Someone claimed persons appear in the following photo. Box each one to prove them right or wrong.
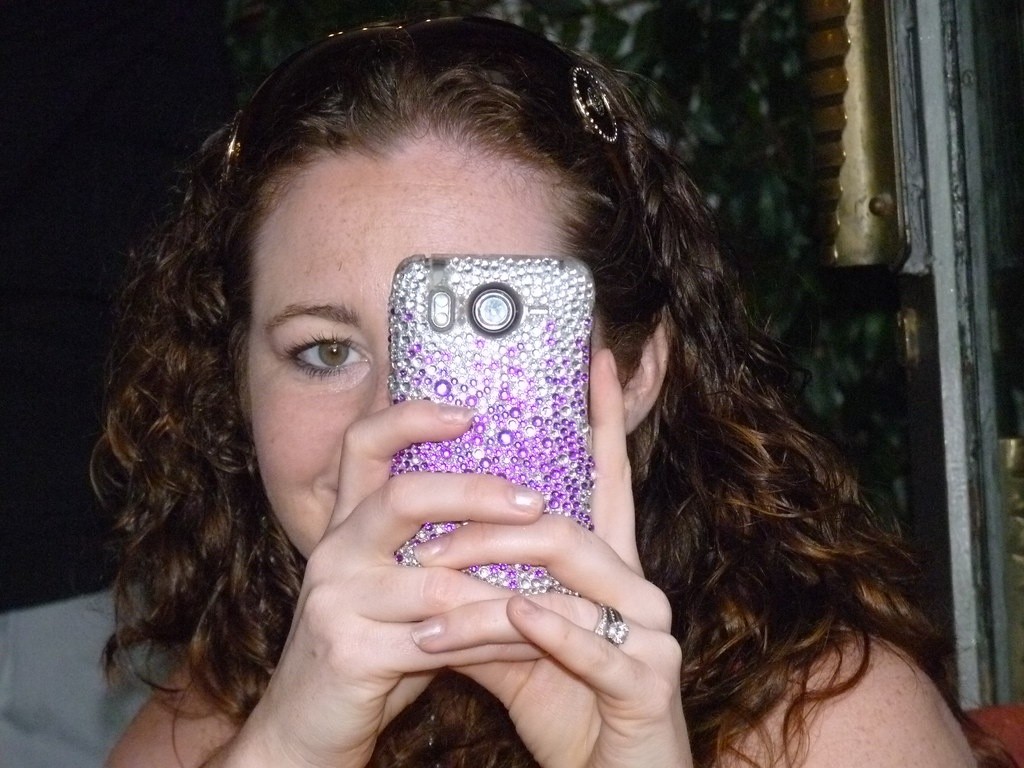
[87,17,979,767]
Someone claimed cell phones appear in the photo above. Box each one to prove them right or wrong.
[387,253,605,640]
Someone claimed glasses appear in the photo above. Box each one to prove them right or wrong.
[223,13,639,227]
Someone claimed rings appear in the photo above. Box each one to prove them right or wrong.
[593,600,629,648]
